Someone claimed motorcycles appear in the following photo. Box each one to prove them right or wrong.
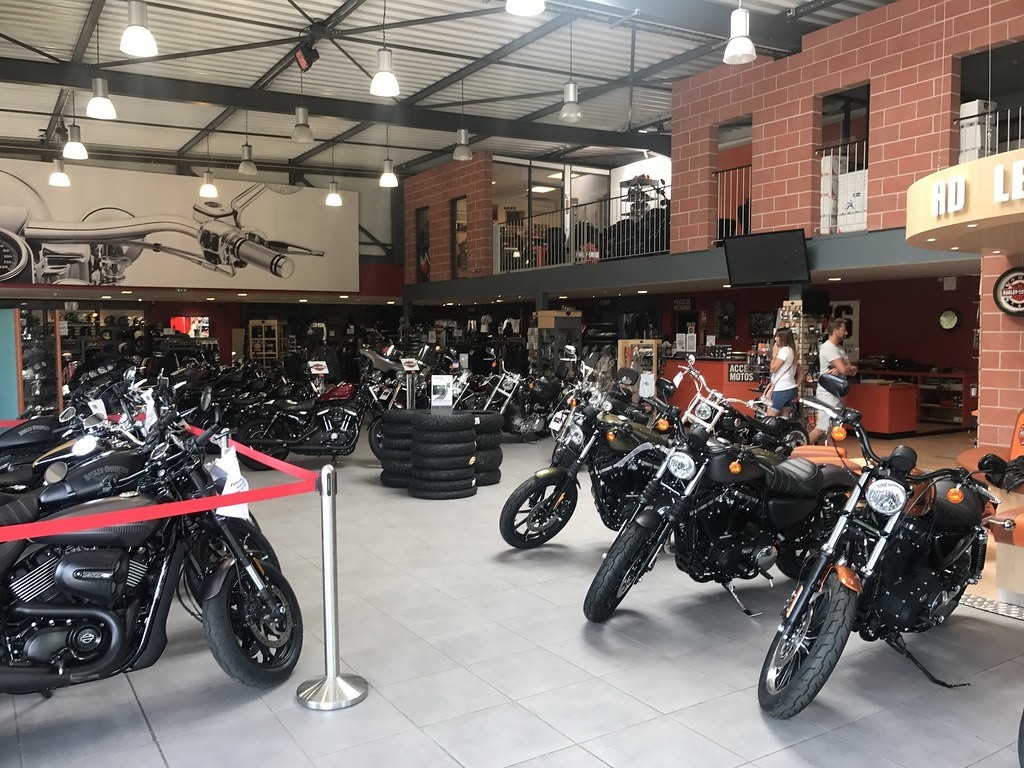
[757,372,1017,720]
[498,344,810,551]
[0,335,600,703]
[0,184,327,288]
[582,352,861,623]
[574,178,671,261]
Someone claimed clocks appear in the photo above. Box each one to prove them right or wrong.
[937,307,961,332]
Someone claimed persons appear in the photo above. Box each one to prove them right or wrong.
[767,327,804,417]
[809,318,857,445]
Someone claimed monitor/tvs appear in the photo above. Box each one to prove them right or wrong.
[723,228,810,288]
[171,316,210,339]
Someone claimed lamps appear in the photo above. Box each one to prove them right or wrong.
[291,70,314,145]
[369,0,400,97]
[295,47,319,72]
[47,25,118,188]
[199,135,218,199]
[325,146,342,206]
[379,124,398,188]
[119,1,158,57]
[504,0,545,17]
[559,21,581,122]
[452,77,475,163]
[238,110,258,176]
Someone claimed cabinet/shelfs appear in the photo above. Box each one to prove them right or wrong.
[857,368,977,429]
[248,319,288,363]
[537,310,582,382]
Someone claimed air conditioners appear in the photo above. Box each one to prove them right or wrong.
[721,0,757,65]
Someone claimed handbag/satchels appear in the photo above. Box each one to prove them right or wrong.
[764,382,776,398]
